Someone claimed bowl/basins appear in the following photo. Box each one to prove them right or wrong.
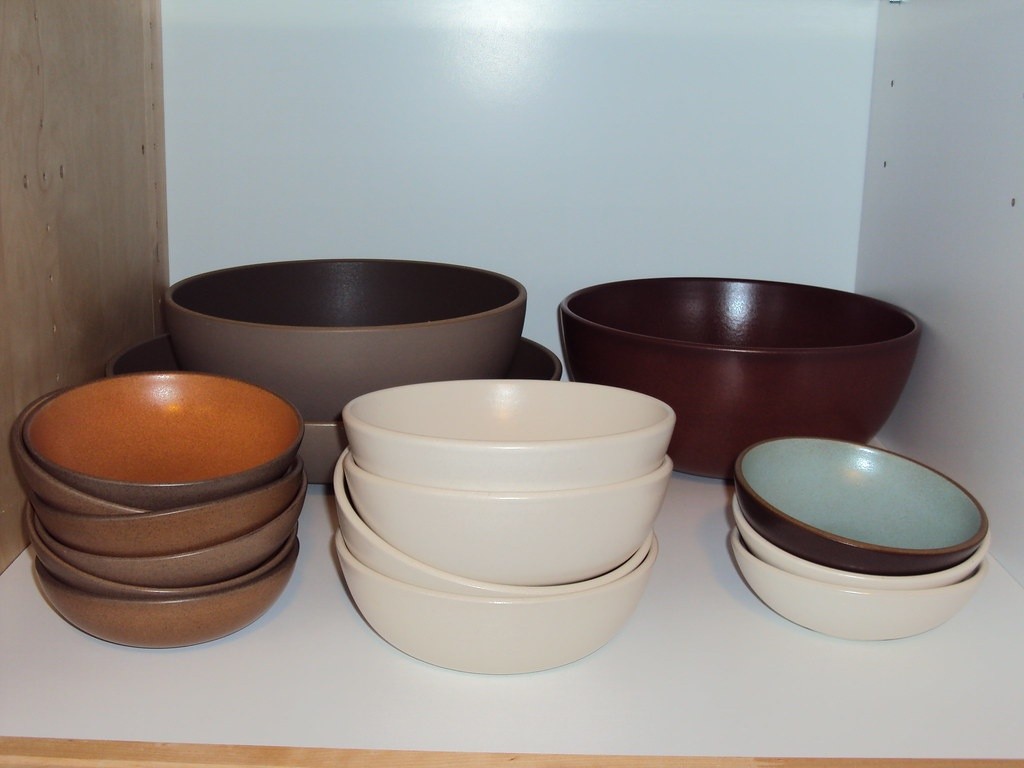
[107,339,562,483]
[162,260,527,423]
[332,379,676,676]
[558,277,919,478]
[8,369,306,648]
[729,437,988,640]
